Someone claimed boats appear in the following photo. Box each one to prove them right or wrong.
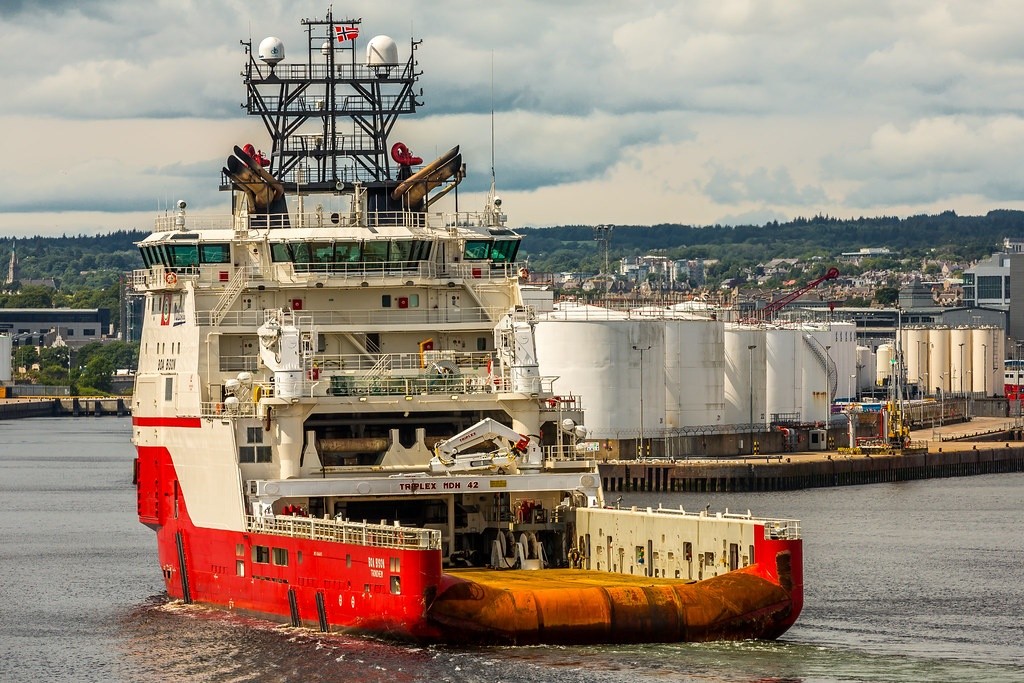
[133,4,803,644]
[1004,359,1024,406]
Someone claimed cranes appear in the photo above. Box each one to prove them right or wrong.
[747,267,839,319]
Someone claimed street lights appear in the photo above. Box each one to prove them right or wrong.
[1017,344,1022,370]
[920,372,928,428]
[826,345,832,450]
[980,343,987,399]
[965,370,972,421]
[923,341,932,396]
[1011,346,1014,369]
[847,374,856,449]
[748,345,758,454]
[632,345,655,456]
[958,343,967,393]
[941,371,949,425]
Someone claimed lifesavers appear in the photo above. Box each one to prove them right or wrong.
[166,273,177,284]
